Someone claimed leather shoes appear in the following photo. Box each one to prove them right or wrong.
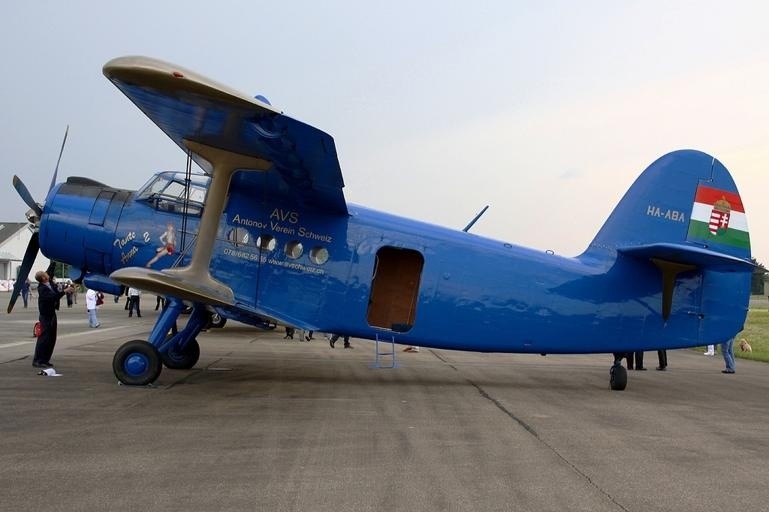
[32,360,54,369]
[721,368,735,373]
[704,352,714,356]
[656,366,668,371]
[403,347,419,353]
[330,339,335,348]
[627,367,647,370]
[344,345,353,349]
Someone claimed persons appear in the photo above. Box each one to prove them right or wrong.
[124,286,131,309]
[625,350,648,370]
[297,328,310,341]
[153,296,165,310]
[329,334,354,349]
[284,327,294,339]
[27,276,32,307]
[31,261,75,368]
[73,284,81,304]
[127,287,144,317]
[85,288,101,329]
[22,280,30,307]
[655,348,667,371]
[403,344,420,352]
[703,344,715,356]
[96,291,104,309]
[720,335,735,373]
[113,295,119,303]
[65,280,74,308]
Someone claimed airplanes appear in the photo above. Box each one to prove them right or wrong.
[8,55,769,384]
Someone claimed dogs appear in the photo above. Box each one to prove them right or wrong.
[739,339,752,352]
